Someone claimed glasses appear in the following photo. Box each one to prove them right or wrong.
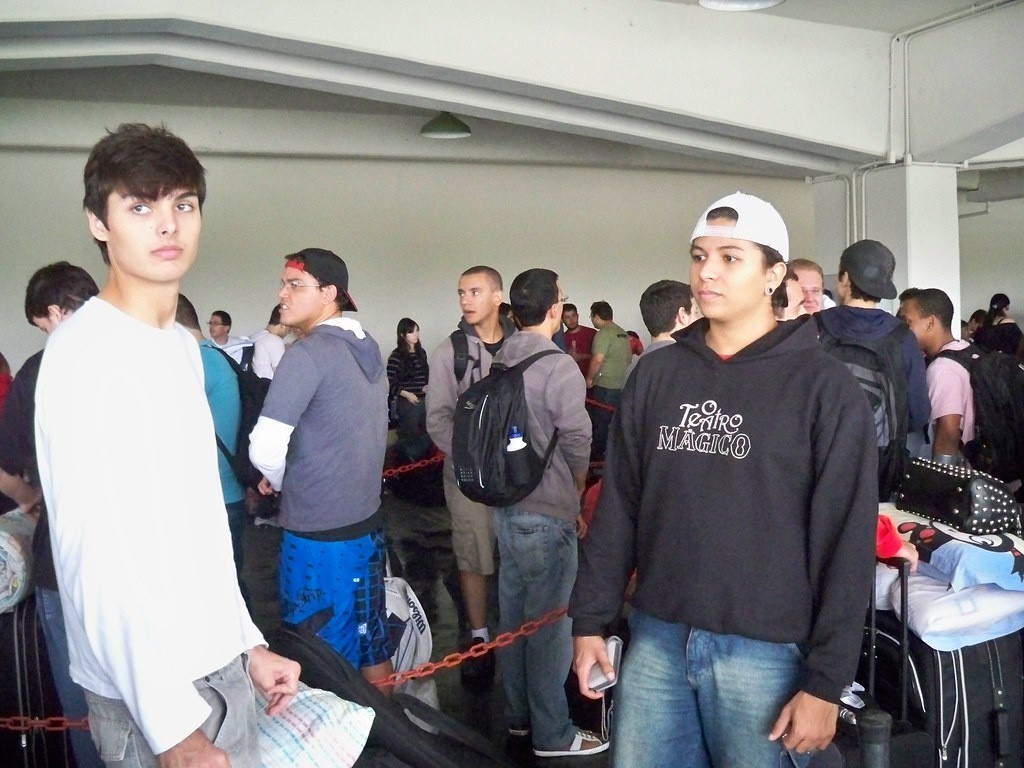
[553,293,569,306]
[278,279,321,289]
[204,321,225,327]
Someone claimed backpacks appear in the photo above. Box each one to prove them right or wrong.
[451,349,571,508]
[927,341,1024,485]
[198,344,273,513]
[810,311,910,504]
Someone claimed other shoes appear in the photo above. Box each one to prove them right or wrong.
[508,723,530,736]
[531,726,610,756]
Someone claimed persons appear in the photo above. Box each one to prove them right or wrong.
[174,293,249,585]
[619,279,704,398]
[425,266,520,694]
[585,301,632,486]
[209,310,255,372]
[489,269,610,757]
[0,262,105,768]
[385,317,430,435]
[247,303,292,383]
[31,121,302,768]
[771,236,1024,573]
[248,248,392,697]
[626,330,644,356]
[568,192,880,768]
[561,303,598,380]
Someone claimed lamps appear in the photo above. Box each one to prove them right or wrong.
[419,111,471,138]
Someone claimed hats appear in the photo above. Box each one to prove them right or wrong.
[689,191,789,307]
[283,248,359,313]
[841,239,897,300]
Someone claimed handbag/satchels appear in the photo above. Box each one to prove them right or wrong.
[894,456,1022,540]
[374,547,444,739]
[860,502,1024,768]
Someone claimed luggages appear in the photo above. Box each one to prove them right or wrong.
[856,553,910,768]
